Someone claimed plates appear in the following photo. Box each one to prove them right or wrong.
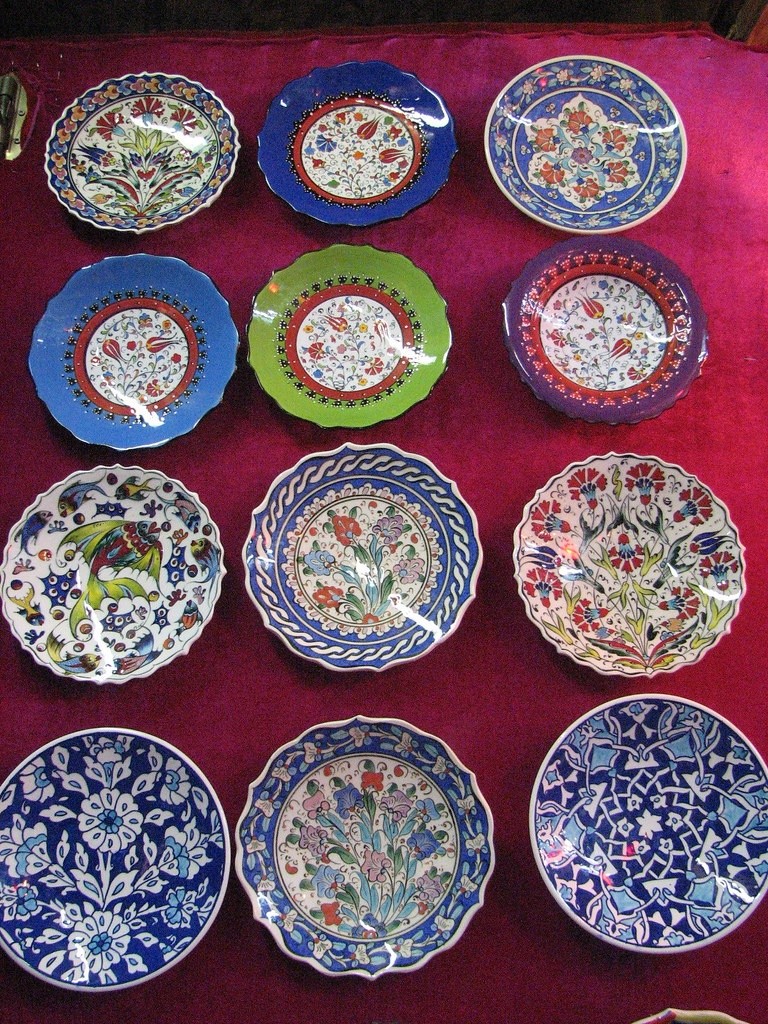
[0,465,227,685]
[28,253,242,453]
[529,693,768,954]
[235,714,496,982]
[513,452,748,678]
[0,727,232,993]
[243,442,483,673]
[502,233,710,426]
[246,241,454,431]
[43,71,242,235]
[484,54,688,234]
[257,61,460,227]
